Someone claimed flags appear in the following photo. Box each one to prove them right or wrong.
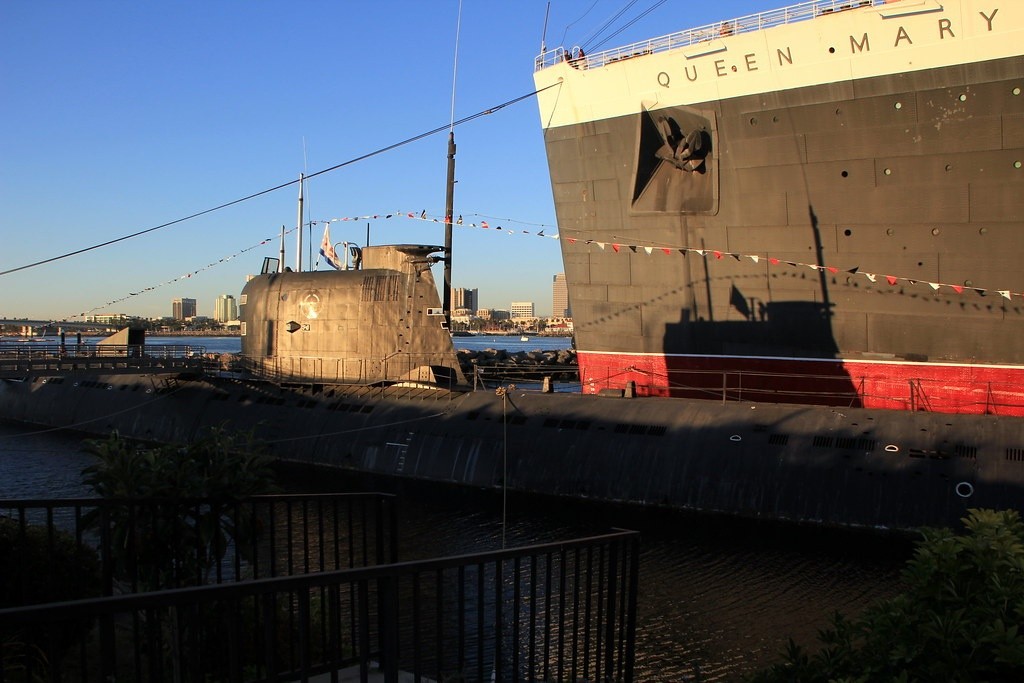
[319,223,342,270]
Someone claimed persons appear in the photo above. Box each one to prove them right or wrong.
[720,22,733,36]
[560,49,586,70]
